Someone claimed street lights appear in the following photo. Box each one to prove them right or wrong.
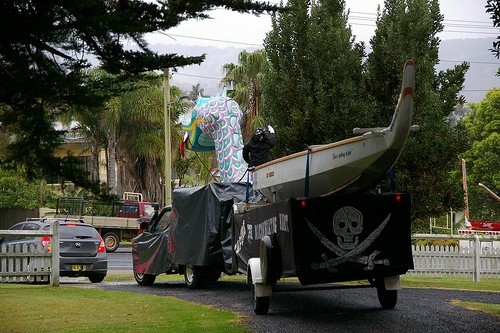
[163,96,190,207]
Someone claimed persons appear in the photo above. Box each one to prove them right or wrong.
[242,124,277,167]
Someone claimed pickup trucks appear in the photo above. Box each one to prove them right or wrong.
[131,186,414,315]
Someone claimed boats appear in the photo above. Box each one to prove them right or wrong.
[176,60,416,200]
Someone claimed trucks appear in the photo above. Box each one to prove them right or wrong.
[46,202,161,253]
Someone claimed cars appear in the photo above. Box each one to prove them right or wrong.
[7,218,108,283]
